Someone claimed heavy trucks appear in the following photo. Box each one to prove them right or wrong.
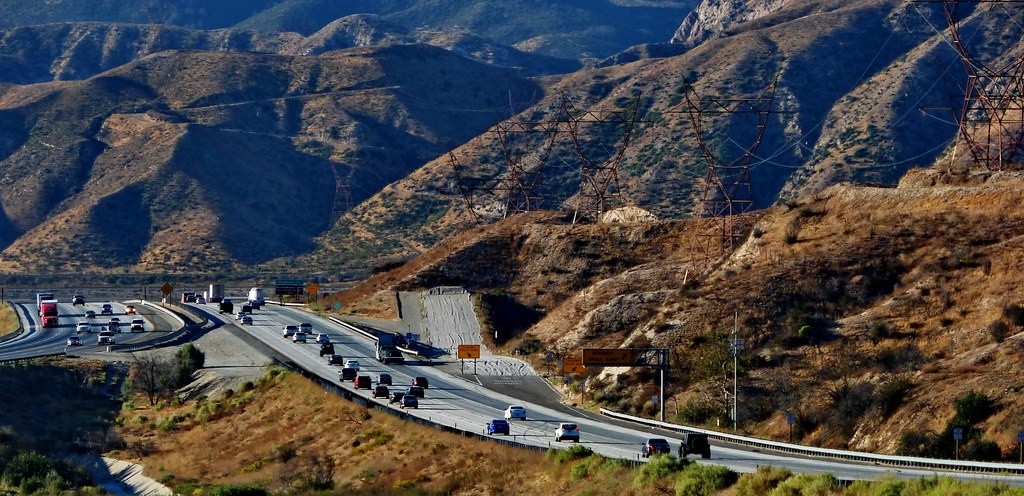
[374,333,404,364]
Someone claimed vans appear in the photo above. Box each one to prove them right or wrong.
[194,292,203,301]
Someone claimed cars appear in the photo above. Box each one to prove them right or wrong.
[505,405,527,421]
[486,419,510,436]
[109,317,122,326]
[195,297,206,305]
[84,310,96,319]
[389,391,406,404]
[399,395,419,409]
[328,354,343,366]
[315,333,330,344]
[240,316,252,325]
[67,336,83,347]
[372,385,390,400]
[235,311,246,321]
[642,438,670,458]
[185,295,196,303]
[376,373,393,385]
[343,360,360,372]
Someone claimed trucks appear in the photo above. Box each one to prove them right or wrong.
[208,284,225,303]
[246,287,264,306]
[180,293,193,303]
[37,291,60,329]
[202,291,209,303]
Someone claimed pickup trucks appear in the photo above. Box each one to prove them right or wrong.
[75,321,91,335]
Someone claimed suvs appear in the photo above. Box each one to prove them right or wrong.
[411,376,429,389]
[240,305,252,314]
[298,323,313,335]
[337,368,358,382]
[96,331,115,346]
[72,295,86,307]
[101,304,114,315]
[319,342,335,357]
[677,433,711,459]
[404,386,424,398]
[282,325,300,338]
[220,298,231,304]
[292,331,306,344]
[130,319,145,334]
[555,422,580,443]
[106,321,123,334]
[353,375,372,390]
[125,305,136,316]
